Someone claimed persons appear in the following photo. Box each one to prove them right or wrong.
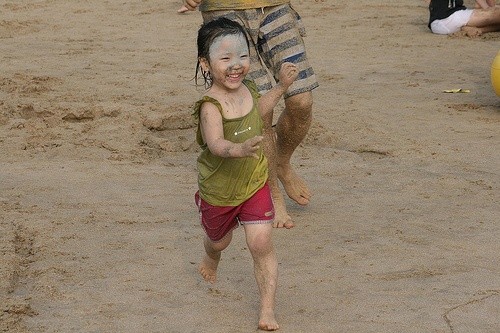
[427,0,500,37]
[194,17,298,331]
[183,0,319,229]
